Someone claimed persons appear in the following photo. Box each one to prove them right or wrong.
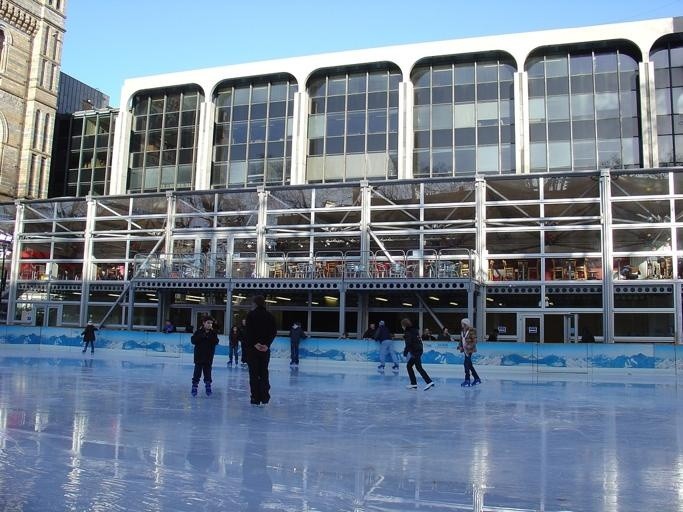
[188,316,220,395]
[226,325,240,365]
[238,318,251,363]
[399,318,435,390]
[373,319,399,369]
[162,313,224,335]
[454,317,481,387]
[240,293,277,405]
[80,320,97,354]
[287,320,307,364]
[362,320,499,342]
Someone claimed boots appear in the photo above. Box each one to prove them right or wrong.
[190,384,199,397]
[392,365,399,370]
[472,377,483,387]
[227,359,246,367]
[204,384,211,397]
[423,381,438,392]
[406,384,418,389]
[288,359,299,366]
[377,365,386,370]
[461,378,471,386]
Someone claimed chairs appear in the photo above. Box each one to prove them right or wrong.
[96,259,682,281]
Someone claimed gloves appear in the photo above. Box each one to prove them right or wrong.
[403,351,408,356]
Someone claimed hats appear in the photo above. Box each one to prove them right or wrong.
[378,320,384,327]
[460,318,470,326]
[87,320,93,325]
